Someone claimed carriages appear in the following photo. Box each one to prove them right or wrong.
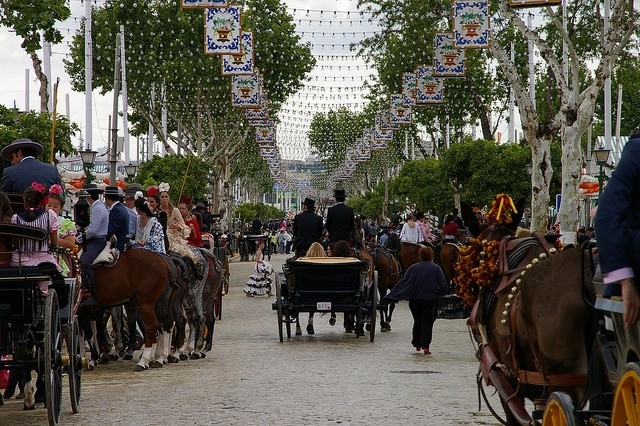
[237,230,272,262]
[272,232,403,343]
[459,194,640,426]
[0,190,85,426]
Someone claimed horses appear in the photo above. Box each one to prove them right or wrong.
[343,228,378,336]
[395,237,460,280]
[95,250,190,365]
[80,246,179,372]
[474,218,600,426]
[293,309,337,336]
[136,248,230,360]
[365,230,402,333]
[121,246,210,361]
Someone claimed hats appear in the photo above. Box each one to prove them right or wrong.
[301,197,316,208]
[104,185,123,195]
[80,183,105,195]
[1,138,45,161]
[333,188,348,197]
[196,202,208,210]
[124,182,145,192]
[123,189,136,199]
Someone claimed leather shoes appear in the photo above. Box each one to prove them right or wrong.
[424,349,432,354]
[416,345,421,351]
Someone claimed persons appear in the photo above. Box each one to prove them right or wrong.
[45,185,76,278]
[324,189,354,244]
[306,241,350,257]
[11,181,63,297]
[0,192,13,268]
[103,186,129,250]
[178,194,203,247]
[294,198,323,257]
[158,183,191,252]
[74,183,109,297]
[146,196,168,251]
[124,191,137,214]
[118,187,135,252]
[356,207,465,251]
[235,213,295,253]
[383,248,449,354]
[595,137,640,331]
[0,138,65,213]
[126,198,166,255]
[242,240,274,297]
[196,202,234,260]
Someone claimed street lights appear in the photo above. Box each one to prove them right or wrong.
[591,143,613,196]
[124,161,136,182]
[78,143,98,185]
[525,162,532,181]
